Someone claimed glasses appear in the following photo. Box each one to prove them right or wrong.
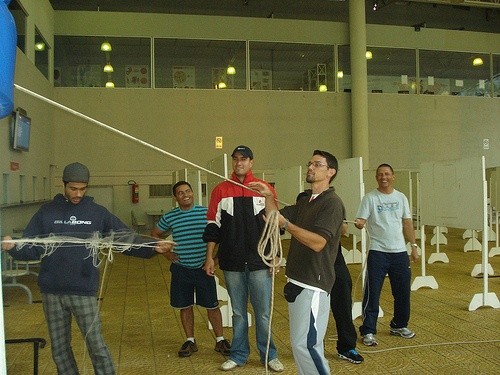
[306,162,330,168]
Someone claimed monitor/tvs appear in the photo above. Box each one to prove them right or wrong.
[13,111,31,152]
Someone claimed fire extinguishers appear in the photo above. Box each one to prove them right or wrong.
[129,180,139,203]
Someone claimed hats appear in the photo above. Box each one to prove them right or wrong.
[63,162,89,183]
[231,145,253,160]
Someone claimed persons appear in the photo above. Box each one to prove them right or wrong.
[294,191,363,363]
[354,163,418,345]
[152,181,231,356]
[248,150,345,375]
[1,162,173,375]
[201,146,284,372]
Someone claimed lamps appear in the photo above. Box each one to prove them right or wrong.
[227,64,236,74]
[338,68,343,78]
[106,81,115,87]
[104,62,114,72]
[366,47,373,59]
[218,81,225,88]
[320,85,328,92]
[100,39,112,52]
[34,41,45,51]
[473,56,483,66]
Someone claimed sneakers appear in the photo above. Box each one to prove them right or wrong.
[178,339,198,357]
[390,326,416,339]
[337,349,364,364]
[215,339,231,355]
[361,333,379,346]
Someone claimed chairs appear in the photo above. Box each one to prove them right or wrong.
[131,211,145,234]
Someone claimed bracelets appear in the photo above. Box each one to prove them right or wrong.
[285,220,289,229]
[411,244,417,248]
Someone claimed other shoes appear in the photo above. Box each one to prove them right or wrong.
[220,359,248,370]
[262,358,283,372]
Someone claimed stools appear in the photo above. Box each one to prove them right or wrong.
[1,260,41,305]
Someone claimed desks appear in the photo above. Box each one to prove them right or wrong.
[147,210,169,229]
